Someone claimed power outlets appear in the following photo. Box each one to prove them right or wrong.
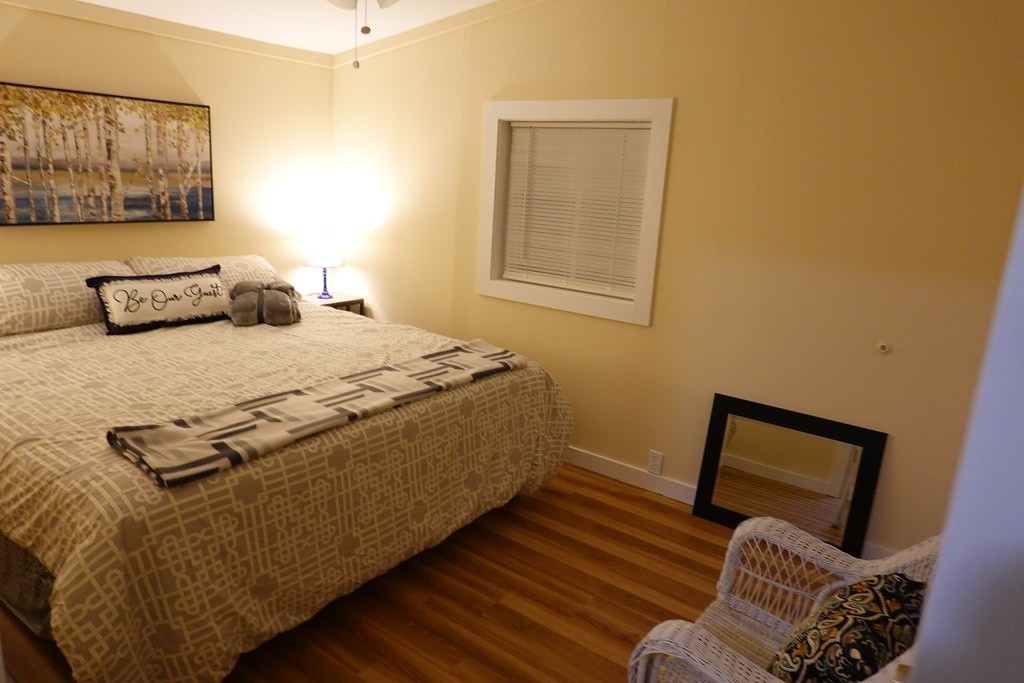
[647,449,663,476]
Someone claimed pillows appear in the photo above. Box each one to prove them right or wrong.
[128,254,301,301]
[0,260,137,336]
[766,570,926,683]
[85,263,232,336]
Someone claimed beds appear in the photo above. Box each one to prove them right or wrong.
[1,256,575,683]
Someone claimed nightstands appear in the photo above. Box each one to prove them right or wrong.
[300,289,364,316]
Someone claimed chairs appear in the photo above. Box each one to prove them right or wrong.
[627,516,946,683]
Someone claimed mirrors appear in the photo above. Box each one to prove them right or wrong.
[693,393,890,562]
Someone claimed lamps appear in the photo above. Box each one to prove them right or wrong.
[328,0,401,69]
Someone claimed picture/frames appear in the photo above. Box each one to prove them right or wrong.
[0,79,214,225]
